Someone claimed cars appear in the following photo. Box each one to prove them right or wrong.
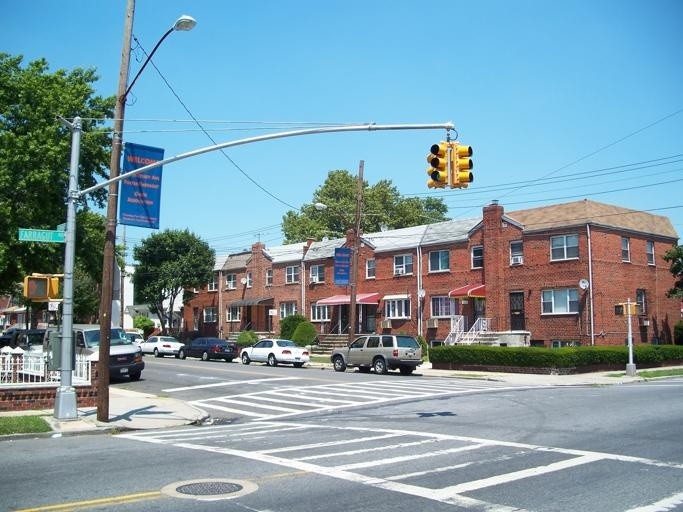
[239,338,309,367]
[0,323,185,382]
[178,337,238,362]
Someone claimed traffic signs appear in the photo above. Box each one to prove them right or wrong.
[17,228,65,244]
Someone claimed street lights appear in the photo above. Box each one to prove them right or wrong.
[315,159,364,342]
[95,3,195,420]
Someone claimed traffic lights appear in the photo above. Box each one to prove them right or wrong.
[426,142,474,191]
[614,303,626,315]
[23,275,49,300]
[629,301,642,315]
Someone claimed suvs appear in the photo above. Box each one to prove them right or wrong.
[329,334,423,375]
